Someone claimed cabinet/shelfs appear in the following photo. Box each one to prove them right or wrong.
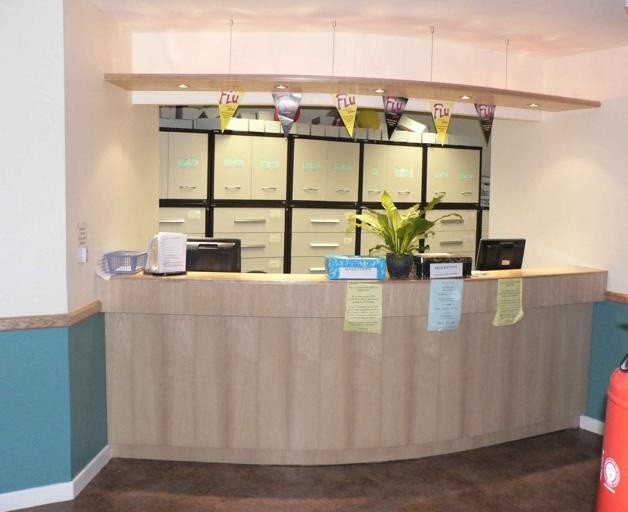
[159,132,209,200]
[423,145,481,208]
[159,204,210,239]
[290,135,361,205]
[359,139,424,208]
[358,210,423,257]
[422,208,480,269]
[211,131,290,204]
[288,210,358,274]
[210,205,289,274]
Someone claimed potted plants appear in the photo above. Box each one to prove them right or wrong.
[344,190,462,279]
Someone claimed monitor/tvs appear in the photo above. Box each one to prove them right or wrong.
[476,239,526,271]
[186,238,241,272]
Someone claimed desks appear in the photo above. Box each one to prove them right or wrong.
[103,266,608,465]
